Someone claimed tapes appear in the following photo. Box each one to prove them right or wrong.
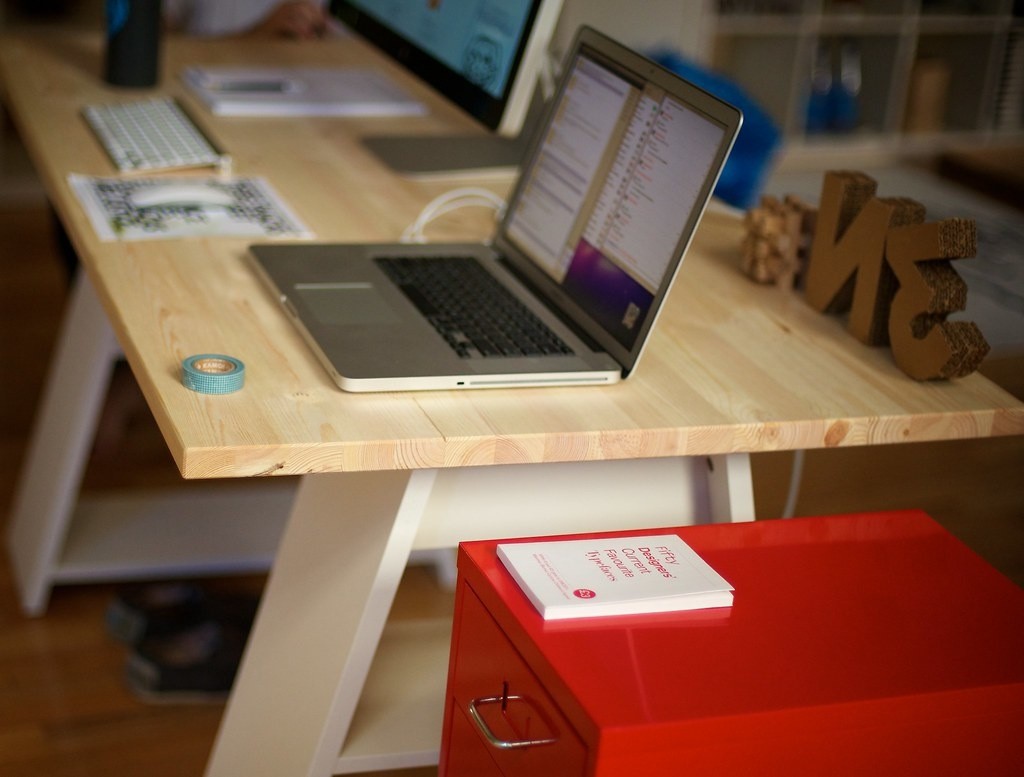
[181,353,246,395]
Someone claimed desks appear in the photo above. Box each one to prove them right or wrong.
[0,1,1024,777]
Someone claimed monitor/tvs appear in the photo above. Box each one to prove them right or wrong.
[326,0,565,178]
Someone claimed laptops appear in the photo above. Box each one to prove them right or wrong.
[247,25,743,393]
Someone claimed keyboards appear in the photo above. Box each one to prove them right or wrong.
[83,92,232,177]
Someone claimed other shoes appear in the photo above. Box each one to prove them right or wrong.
[105,582,261,706]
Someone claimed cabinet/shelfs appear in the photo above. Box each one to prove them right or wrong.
[685,0,1023,168]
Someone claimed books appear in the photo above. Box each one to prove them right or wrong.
[495,533,736,620]
[172,66,429,119]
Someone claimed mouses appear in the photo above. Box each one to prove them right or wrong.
[127,182,236,212]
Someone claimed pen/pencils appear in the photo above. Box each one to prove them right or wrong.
[201,77,296,92]
